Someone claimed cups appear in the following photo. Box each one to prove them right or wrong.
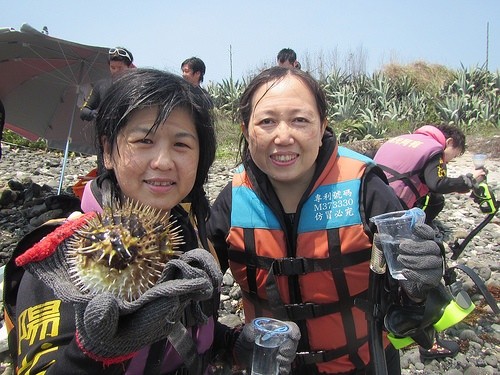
[251,318,293,375]
[369,209,426,280]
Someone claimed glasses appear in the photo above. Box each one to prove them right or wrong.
[109,48,131,61]
[469,176,499,215]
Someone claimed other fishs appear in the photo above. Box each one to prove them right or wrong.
[65,195,187,304]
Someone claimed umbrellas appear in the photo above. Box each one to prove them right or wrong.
[0,23,137,196]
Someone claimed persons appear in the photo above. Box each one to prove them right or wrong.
[278,48,301,68]
[204,67,446,375]
[3,68,301,375]
[372,124,486,226]
[80,47,133,176]
[181,56,206,89]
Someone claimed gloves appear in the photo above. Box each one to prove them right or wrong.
[373,223,444,302]
[232,319,301,375]
[74,277,213,358]
